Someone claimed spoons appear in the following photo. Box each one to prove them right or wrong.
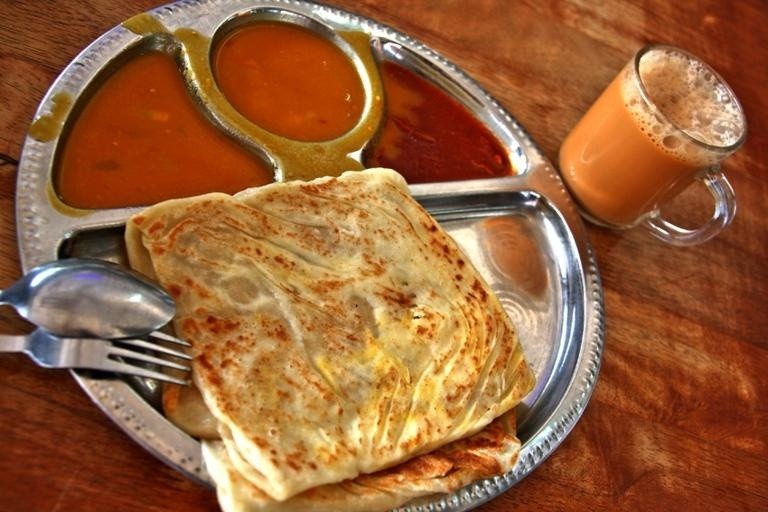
[0,256,180,340]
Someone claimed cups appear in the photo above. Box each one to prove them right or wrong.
[559,43,750,248]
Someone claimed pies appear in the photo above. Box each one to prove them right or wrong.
[124,166,536,512]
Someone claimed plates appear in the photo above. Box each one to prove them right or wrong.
[16,0,602,512]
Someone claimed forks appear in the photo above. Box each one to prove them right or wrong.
[1,327,194,389]
